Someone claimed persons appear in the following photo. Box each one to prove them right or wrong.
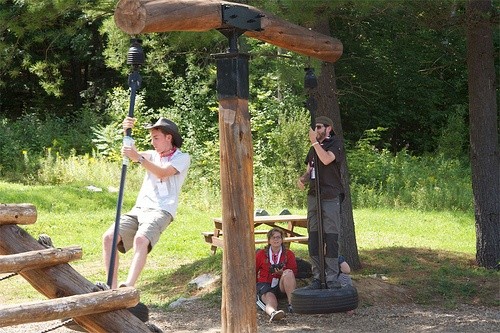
[92,117,191,291]
[256,229,296,323]
[300,116,340,289]
[323,241,354,287]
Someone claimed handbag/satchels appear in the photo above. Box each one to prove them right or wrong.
[294,259,313,278]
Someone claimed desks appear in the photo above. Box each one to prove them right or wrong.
[204,214,308,256]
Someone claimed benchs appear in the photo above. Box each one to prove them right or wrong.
[254,237,309,244]
[201,230,269,236]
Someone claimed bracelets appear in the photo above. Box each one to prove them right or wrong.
[137,156,144,163]
[311,142,319,146]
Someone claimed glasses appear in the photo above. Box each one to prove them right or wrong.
[270,235,281,240]
[315,125,327,130]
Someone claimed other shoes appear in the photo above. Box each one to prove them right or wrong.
[327,281,340,288]
[270,310,284,322]
[95,281,110,291]
[119,283,126,288]
[288,306,293,313]
[308,278,321,288]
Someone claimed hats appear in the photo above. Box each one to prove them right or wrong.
[143,117,182,149]
[316,116,336,137]
